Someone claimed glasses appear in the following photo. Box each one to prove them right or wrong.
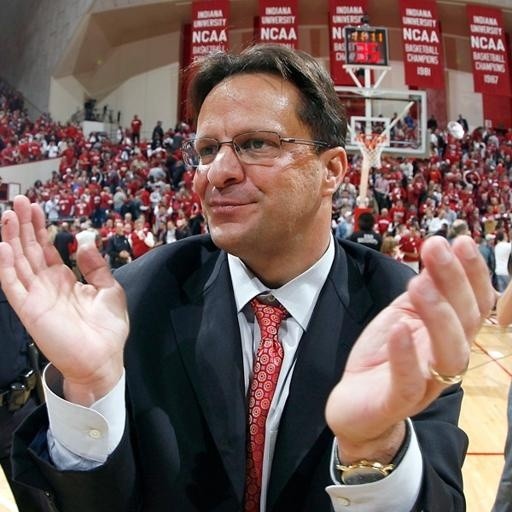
[178,130,334,169]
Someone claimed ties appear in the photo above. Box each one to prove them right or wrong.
[241,295,291,511]
